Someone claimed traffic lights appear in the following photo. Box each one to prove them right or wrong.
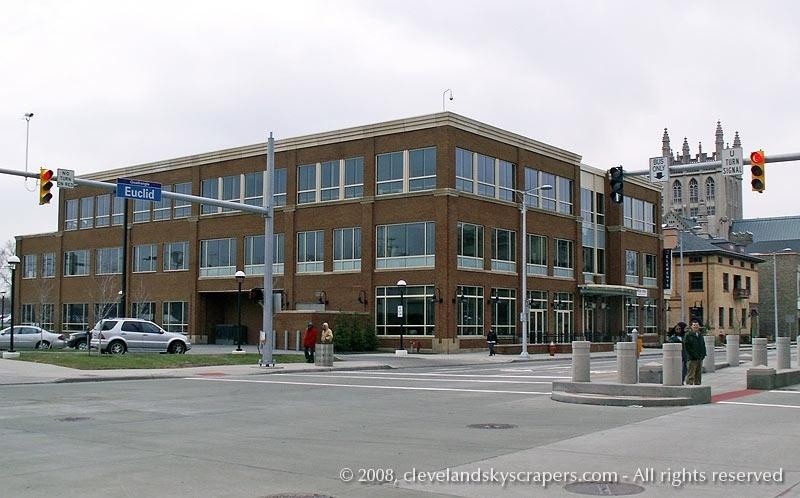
[609,165,623,203]
[750,150,765,191]
[40,166,54,205]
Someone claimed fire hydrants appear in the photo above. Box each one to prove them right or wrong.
[412,338,421,352]
[547,339,556,357]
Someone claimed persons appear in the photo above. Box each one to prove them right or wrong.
[320,321,334,344]
[486,328,497,358]
[303,321,318,363]
[664,320,687,386]
[682,319,707,386]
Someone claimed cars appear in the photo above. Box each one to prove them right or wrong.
[0,313,8,318]
[0,325,65,351]
[1,314,11,323]
[66,332,94,350]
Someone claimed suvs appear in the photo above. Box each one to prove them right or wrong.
[90,317,192,354]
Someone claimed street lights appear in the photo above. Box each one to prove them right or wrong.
[442,88,453,111]
[235,270,246,350]
[395,278,407,349]
[678,225,703,321]
[0,291,5,329]
[519,183,553,358]
[8,253,20,352]
[772,248,792,341]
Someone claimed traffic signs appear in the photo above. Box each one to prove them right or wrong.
[720,146,744,176]
[56,169,75,189]
[115,177,163,203]
[649,156,669,183]
